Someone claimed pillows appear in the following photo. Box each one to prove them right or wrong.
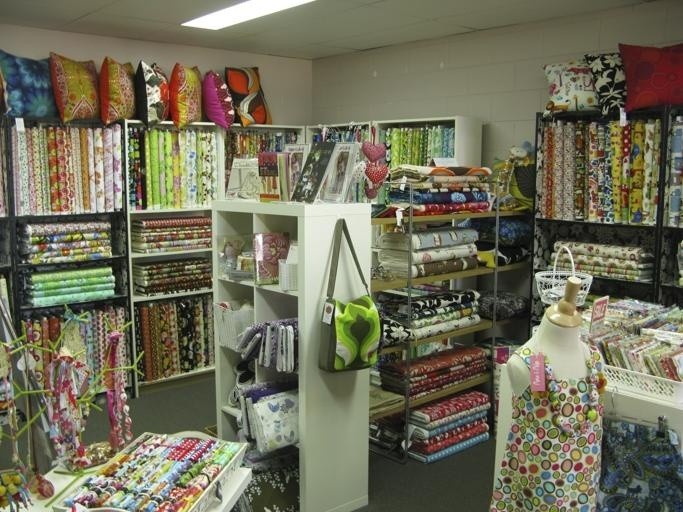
[170,63,202,130]
[618,41,683,107]
[202,69,235,130]
[543,58,597,110]
[134,60,170,131]
[0,49,58,119]
[50,52,100,124]
[225,66,273,127]
[97,56,134,127]
[583,52,623,108]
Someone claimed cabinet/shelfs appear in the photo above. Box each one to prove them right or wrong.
[2,120,139,401]
[373,116,458,199]
[307,121,372,202]
[531,109,661,335]
[128,118,227,390]
[224,123,305,203]
[663,106,682,307]
[212,200,370,512]
[373,208,531,465]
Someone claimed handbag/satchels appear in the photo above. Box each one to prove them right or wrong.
[319,218,384,373]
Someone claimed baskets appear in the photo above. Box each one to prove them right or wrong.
[641,308,683,338]
[534,246,594,307]
[596,332,683,403]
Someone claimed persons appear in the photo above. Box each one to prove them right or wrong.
[330,152,346,194]
[490,277,608,508]
